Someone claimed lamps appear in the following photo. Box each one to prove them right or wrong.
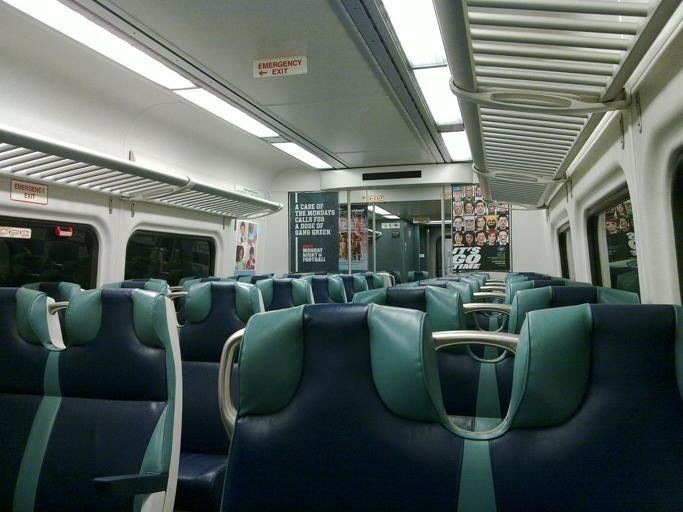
[0,0,336,172]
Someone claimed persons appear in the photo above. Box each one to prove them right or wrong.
[338,232,362,260]
[236,221,256,271]
[453,185,510,247]
[604,202,633,260]
